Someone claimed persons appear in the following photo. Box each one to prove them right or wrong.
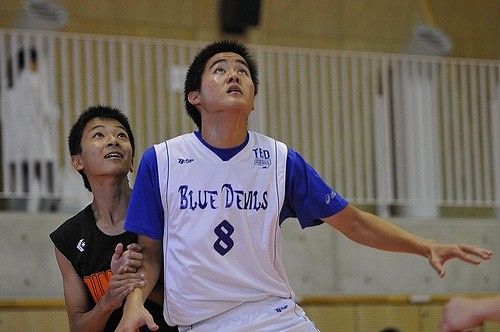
[115,39,493,332]
[440,294,500,332]
[47,106,178,332]
[4,48,63,211]
